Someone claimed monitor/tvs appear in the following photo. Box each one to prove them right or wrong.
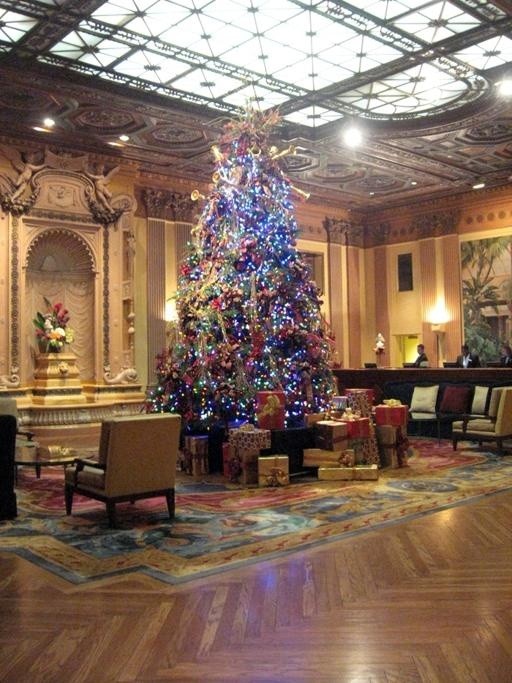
[443,363,458,368]
[364,363,376,369]
[402,363,415,367]
[486,361,501,367]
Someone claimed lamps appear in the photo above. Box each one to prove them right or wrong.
[430,323,446,368]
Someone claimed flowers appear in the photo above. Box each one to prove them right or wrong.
[31,296,76,352]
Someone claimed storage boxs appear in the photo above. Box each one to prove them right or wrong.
[182,389,409,488]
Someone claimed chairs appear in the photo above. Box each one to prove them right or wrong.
[0,414,18,522]
[452,385,512,456]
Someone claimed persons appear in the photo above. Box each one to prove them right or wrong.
[0,144,53,204]
[455,344,482,368]
[413,343,429,367]
[83,161,121,215]
[500,346,512,366]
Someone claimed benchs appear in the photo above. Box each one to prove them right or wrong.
[381,379,472,446]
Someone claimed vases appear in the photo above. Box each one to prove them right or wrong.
[39,340,61,353]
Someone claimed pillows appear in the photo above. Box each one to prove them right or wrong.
[487,387,503,419]
[408,383,470,415]
[62,411,181,530]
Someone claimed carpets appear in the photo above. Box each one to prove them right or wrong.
[0,434,512,585]
